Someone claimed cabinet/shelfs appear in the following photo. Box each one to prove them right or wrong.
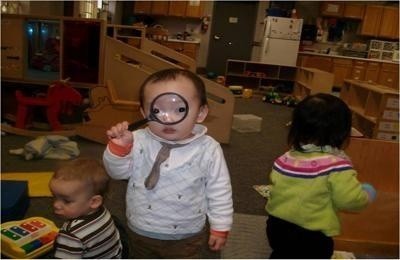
[127,38,199,69]
[169,0,201,18]
[359,1,399,41]
[224,58,336,103]
[321,1,366,20]
[338,78,400,139]
[133,0,168,16]
[302,56,398,88]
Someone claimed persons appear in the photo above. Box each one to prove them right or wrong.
[48,157,123,259]
[103,70,234,259]
[265,93,376,259]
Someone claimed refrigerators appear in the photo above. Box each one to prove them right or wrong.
[261,15,304,66]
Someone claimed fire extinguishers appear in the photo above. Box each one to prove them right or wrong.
[201,15,210,31]
